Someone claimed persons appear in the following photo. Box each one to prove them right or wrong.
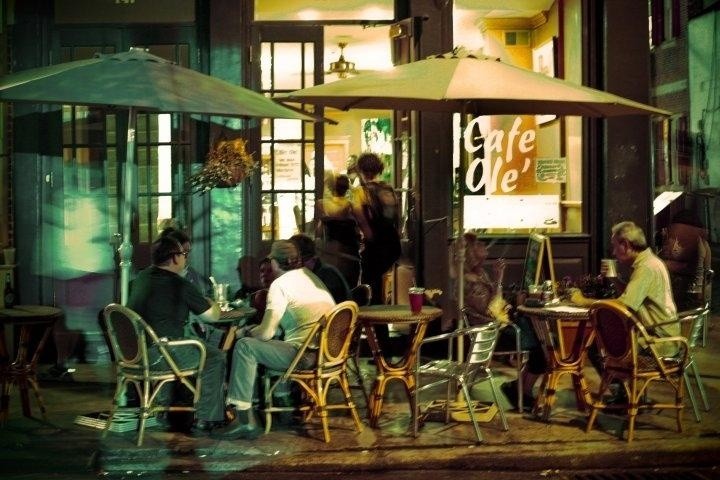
[568,221,682,414]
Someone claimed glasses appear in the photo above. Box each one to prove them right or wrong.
[173,252,188,258]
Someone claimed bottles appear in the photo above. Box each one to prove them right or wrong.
[524,280,561,307]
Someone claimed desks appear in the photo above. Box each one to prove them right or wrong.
[0,305,61,426]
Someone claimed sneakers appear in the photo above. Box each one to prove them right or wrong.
[499,380,536,410]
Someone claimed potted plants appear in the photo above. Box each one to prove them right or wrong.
[182,113,258,196]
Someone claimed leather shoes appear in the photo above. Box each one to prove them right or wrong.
[211,421,262,441]
[190,416,215,437]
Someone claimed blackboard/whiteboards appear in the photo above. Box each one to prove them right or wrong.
[520,233,545,349]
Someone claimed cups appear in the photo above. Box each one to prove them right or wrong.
[408,286,424,314]
[212,282,229,302]
[602,259,617,278]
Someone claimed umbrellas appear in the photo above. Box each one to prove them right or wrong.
[274,46,675,298]
[0,46,339,404]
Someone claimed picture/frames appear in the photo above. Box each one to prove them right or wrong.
[531,36,557,126]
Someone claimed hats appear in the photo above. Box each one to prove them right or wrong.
[264,239,300,259]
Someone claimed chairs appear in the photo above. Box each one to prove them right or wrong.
[294,204,311,234]
[97,276,713,445]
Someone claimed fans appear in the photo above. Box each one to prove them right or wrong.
[294,41,370,79]
